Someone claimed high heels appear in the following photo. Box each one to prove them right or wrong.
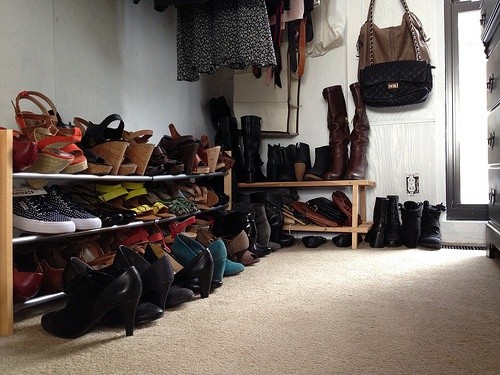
[0,126,75,190]
[75,114,130,176]
[118,155,138,175]
[24,118,88,173]
[14,202,295,340]
[48,110,113,175]
[122,131,156,175]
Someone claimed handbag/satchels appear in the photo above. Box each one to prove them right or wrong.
[356,0,431,69]
[307,198,349,226]
[332,191,362,227]
[358,0,435,108]
[292,201,338,227]
[13,89,58,133]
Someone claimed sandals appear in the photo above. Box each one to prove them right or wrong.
[13,181,230,235]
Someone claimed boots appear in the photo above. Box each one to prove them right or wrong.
[368,198,389,248]
[205,96,332,184]
[345,83,370,180]
[398,201,423,249]
[421,201,445,249]
[387,195,403,247]
[322,85,351,180]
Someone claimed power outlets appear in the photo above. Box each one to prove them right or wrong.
[406,175,420,194]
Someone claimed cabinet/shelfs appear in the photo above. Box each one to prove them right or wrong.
[0,128,232,338]
[233,23,301,135]
[478,0,500,257]
[238,180,376,250]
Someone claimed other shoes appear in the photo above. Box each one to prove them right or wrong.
[298,236,327,248]
[332,232,363,248]
[144,124,234,176]
